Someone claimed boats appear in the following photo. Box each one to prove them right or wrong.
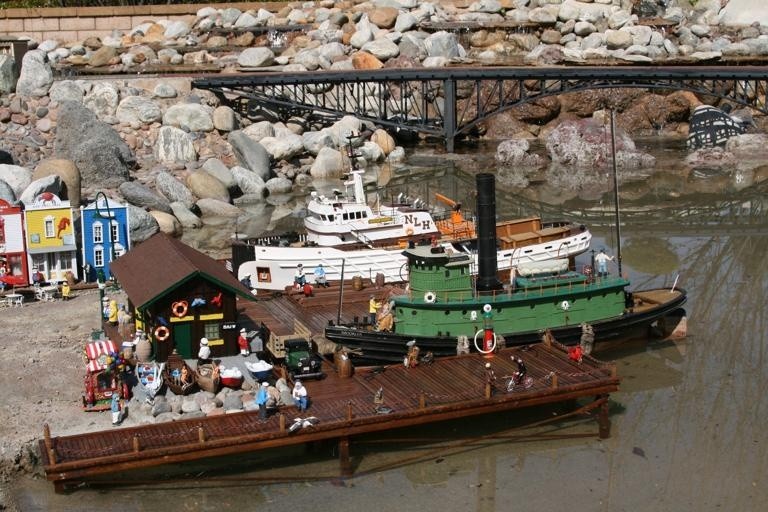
[135,355,274,401]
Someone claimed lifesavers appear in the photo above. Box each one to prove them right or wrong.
[154,326,169,341]
[173,302,187,317]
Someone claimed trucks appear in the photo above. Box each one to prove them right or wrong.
[260,317,323,382]
